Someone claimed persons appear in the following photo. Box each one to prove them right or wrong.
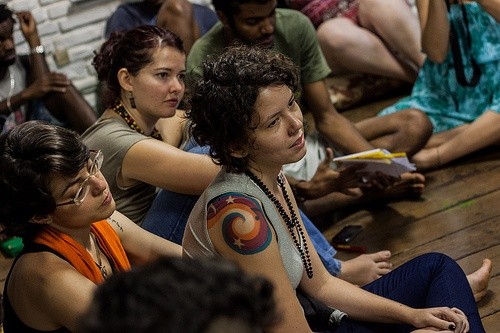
[0,0,500,333]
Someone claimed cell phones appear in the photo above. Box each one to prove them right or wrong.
[331,224,363,245]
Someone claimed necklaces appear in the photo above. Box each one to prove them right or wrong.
[234,158,297,227]
[109,99,163,141]
[244,169,313,278]
[0,63,15,95]
[93,235,109,279]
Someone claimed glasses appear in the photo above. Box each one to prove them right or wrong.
[57,148,104,206]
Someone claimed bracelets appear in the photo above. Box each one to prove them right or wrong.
[6,95,14,113]
[29,46,44,54]
